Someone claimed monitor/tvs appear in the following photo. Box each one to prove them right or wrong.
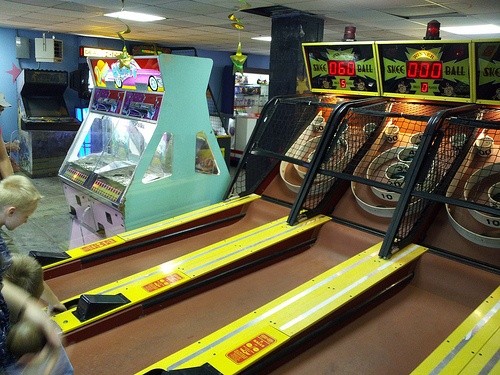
[20,94,71,117]
